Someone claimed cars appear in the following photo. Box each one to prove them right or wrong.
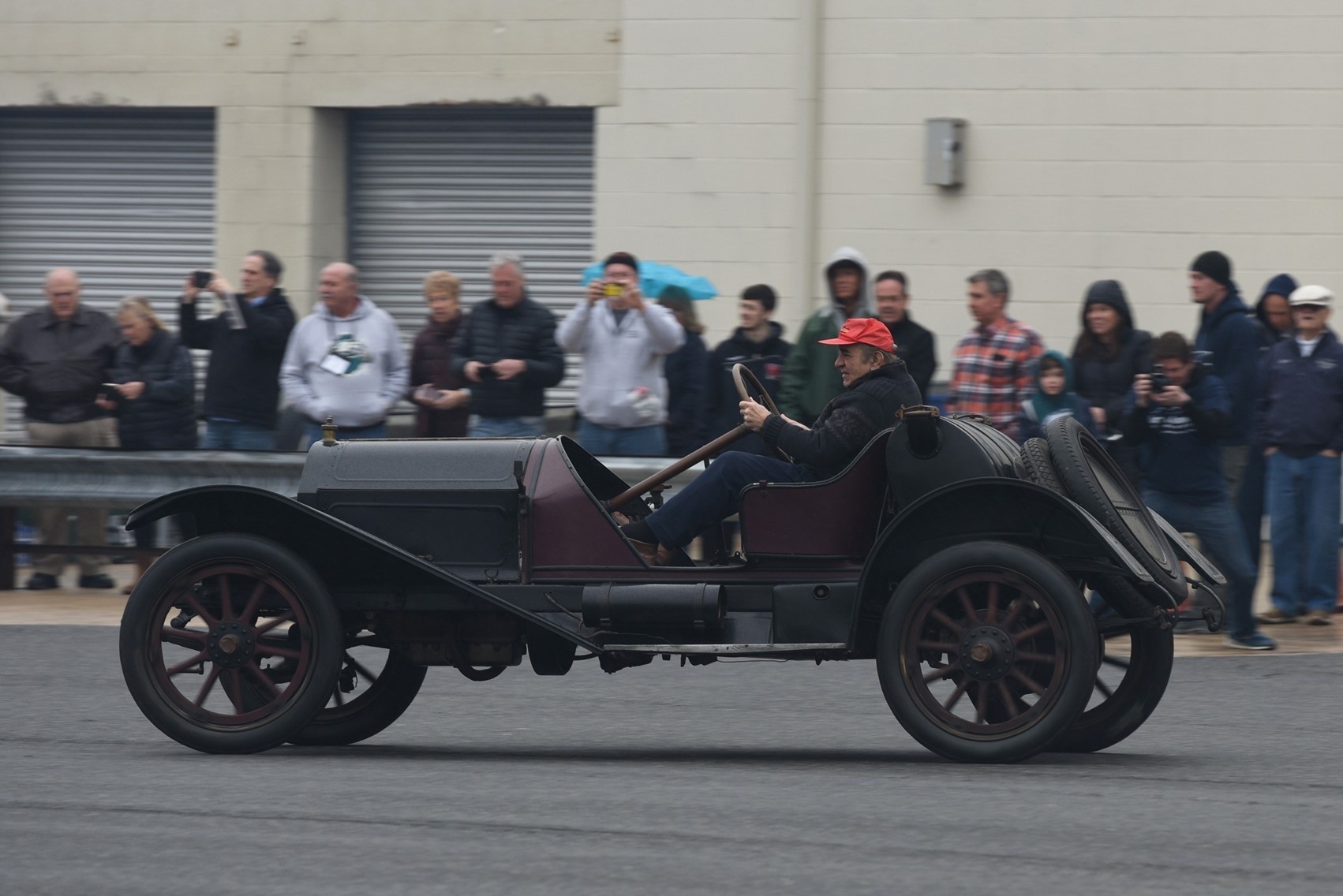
[113,358,1222,760]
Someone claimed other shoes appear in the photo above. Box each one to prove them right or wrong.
[626,537,659,566]
[612,511,671,566]
[80,575,116,588]
[1306,611,1331,624]
[26,572,57,590]
[1252,607,1296,624]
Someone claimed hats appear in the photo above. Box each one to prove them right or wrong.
[605,251,637,271]
[1287,284,1334,309]
[818,317,897,352]
[1192,251,1230,285]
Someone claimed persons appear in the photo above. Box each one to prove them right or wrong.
[172,248,297,455]
[1244,268,1317,604]
[444,251,567,443]
[612,316,925,563]
[1102,326,1278,651]
[706,279,799,464]
[871,268,938,396]
[91,293,199,594]
[1013,345,1095,453]
[1250,283,1343,628]
[0,265,128,592]
[277,261,412,454]
[1172,249,1268,635]
[777,245,885,441]
[402,268,473,441]
[1062,276,1170,625]
[943,264,1050,449]
[551,251,684,460]
[647,283,722,462]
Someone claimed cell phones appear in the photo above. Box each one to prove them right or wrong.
[102,382,118,401]
[601,282,626,298]
[477,365,493,378]
[191,270,216,287]
[423,387,442,401]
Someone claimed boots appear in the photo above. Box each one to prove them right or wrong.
[122,553,154,594]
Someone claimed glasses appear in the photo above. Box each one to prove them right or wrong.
[605,273,637,280]
[1290,305,1322,312]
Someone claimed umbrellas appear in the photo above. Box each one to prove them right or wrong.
[578,260,719,305]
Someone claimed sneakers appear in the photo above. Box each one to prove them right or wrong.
[1223,630,1277,651]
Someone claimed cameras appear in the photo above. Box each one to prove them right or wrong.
[1148,364,1172,394]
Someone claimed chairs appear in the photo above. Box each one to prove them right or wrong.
[736,428,896,565]
